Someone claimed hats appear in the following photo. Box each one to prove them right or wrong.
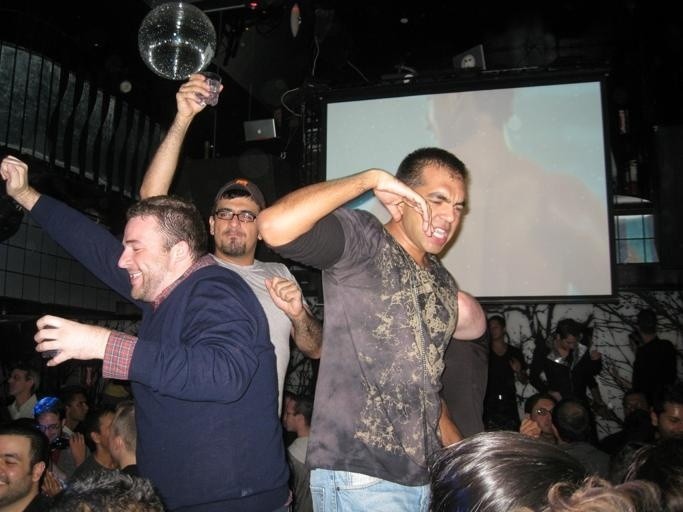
[214,176,266,212]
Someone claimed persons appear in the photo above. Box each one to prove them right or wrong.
[34,397,92,476]
[435,287,491,447]
[284,398,315,512]
[0,423,65,512]
[61,390,90,434]
[632,310,677,403]
[519,392,558,440]
[256,146,467,512]
[528,317,602,403]
[107,399,137,476]
[651,397,683,441]
[604,410,655,479]
[425,432,682,512]
[483,315,528,433]
[623,387,651,424]
[0,155,293,511]
[66,407,119,494]
[139,72,324,421]
[549,393,612,481]
[0,364,40,419]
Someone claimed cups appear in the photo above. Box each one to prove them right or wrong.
[203,72,221,106]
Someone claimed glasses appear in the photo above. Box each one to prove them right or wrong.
[530,406,556,418]
[207,209,257,223]
[37,424,60,431]
